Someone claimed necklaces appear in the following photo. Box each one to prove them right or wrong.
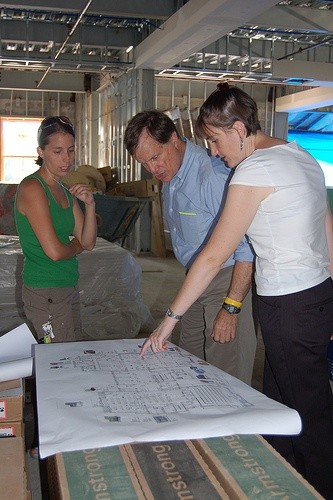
[45,171,70,191]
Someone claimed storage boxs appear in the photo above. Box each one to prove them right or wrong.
[0,379,32,500]
[117,178,160,198]
[46,435,325,500]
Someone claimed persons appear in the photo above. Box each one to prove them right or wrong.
[14,114,97,344]
[140,82,333,500]
[125,110,258,387]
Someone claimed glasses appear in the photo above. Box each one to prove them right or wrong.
[41,116,73,130]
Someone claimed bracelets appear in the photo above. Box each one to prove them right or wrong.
[166,308,183,319]
[225,297,243,308]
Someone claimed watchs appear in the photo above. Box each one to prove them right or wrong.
[222,303,241,314]
